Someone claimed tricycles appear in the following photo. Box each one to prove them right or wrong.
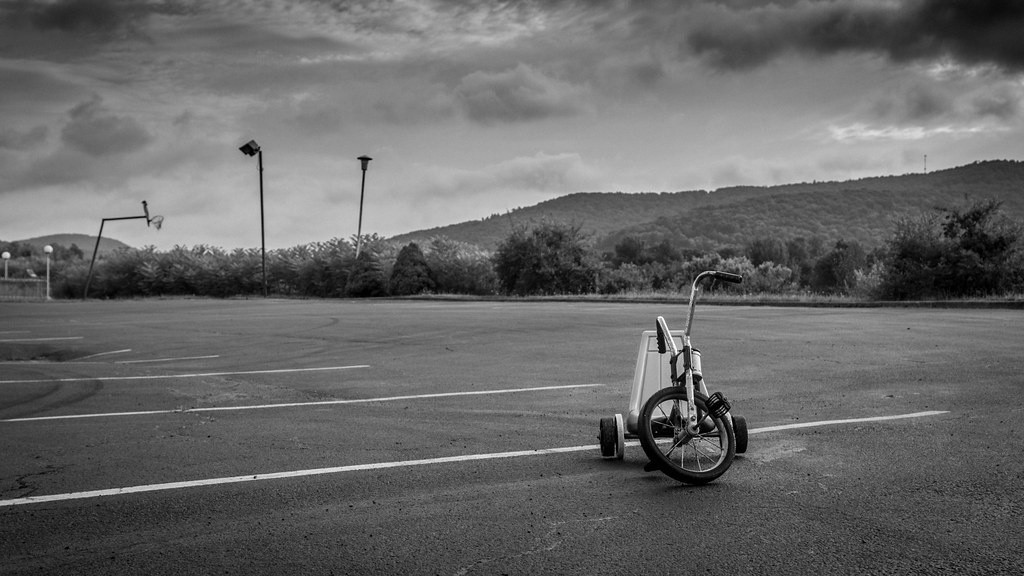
[595,269,749,490]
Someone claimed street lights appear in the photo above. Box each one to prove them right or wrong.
[239,140,267,298]
[41,243,55,300]
[2,251,12,280]
[355,153,373,259]
